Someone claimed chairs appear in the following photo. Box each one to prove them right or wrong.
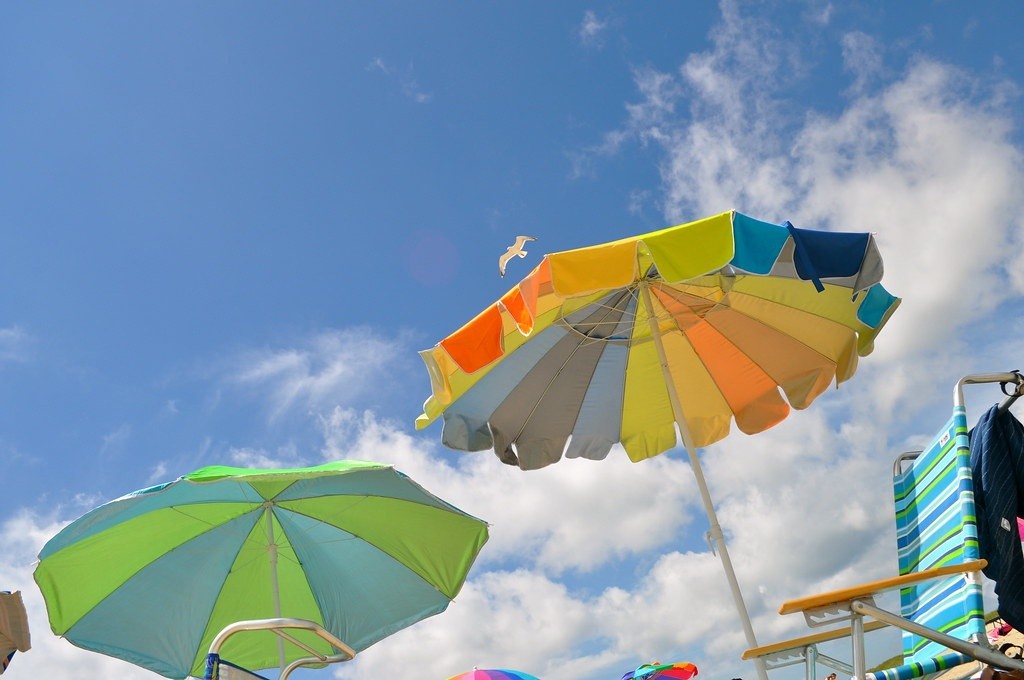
[742,370,1024,680]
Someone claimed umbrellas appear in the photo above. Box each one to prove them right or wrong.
[620,662,700,679]
[415,205,904,678]
[32,459,494,680]
[448,665,540,680]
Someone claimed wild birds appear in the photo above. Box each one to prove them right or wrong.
[498,235,538,279]
